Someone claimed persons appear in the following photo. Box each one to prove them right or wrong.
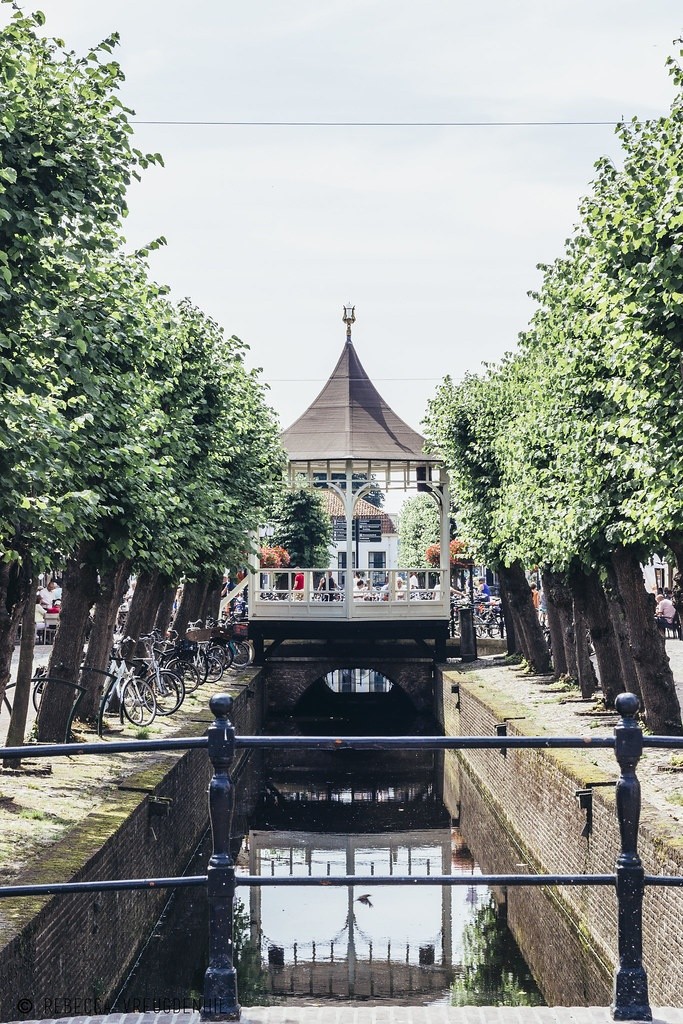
[293,566,304,601]
[33,574,248,642]
[353,572,548,628]
[316,570,343,601]
[647,583,682,639]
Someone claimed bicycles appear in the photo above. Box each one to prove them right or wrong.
[33,584,507,727]
[538,609,552,647]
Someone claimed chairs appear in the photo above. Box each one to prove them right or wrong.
[658,612,678,640]
[20,623,51,645]
[44,613,59,644]
